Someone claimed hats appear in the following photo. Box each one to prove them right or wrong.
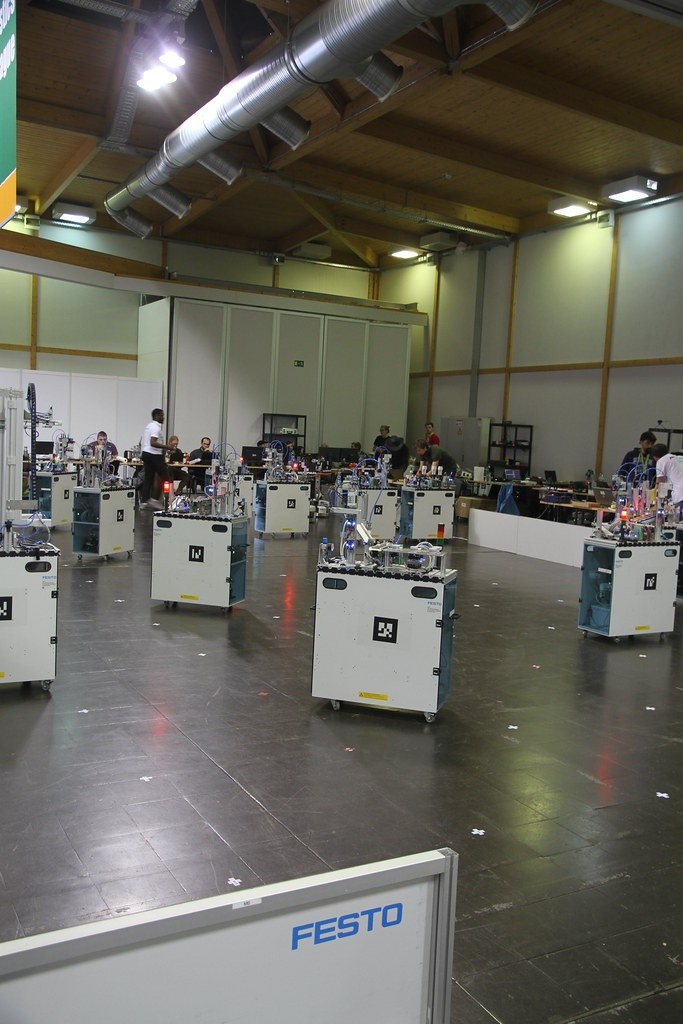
[386,435,405,451]
[257,441,268,446]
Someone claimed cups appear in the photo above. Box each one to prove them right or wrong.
[124,451,132,462]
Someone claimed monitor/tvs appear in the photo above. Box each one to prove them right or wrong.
[545,470,557,489]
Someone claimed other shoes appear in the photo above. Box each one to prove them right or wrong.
[175,488,182,496]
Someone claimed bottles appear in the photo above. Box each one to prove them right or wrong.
[318,537,329,565]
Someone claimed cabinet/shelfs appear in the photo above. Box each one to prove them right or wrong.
[486,422,532,481]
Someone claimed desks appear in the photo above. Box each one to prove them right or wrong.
[541,498,618,524]
[530,486,595,499]
[74,460,291,486]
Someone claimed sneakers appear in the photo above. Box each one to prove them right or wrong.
[139,503,151,509]
[147,498,163,509]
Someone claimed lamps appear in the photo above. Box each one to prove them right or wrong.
[546,196,598,220]
[51,202,97,225]
[600,176,662,206]
[137,30,187,92]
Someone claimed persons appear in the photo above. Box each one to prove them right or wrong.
[139,408,175,511]
[185,437,219,495]
[257,423,462,499]
[618,432,657,490]
[87,431,119,482]
[164,436,191,496]
[652,443,683,564]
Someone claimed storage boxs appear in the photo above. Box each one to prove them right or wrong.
[456,495,497,519]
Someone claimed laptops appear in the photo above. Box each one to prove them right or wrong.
[242,445,263,461]
[592,486,616,506]
